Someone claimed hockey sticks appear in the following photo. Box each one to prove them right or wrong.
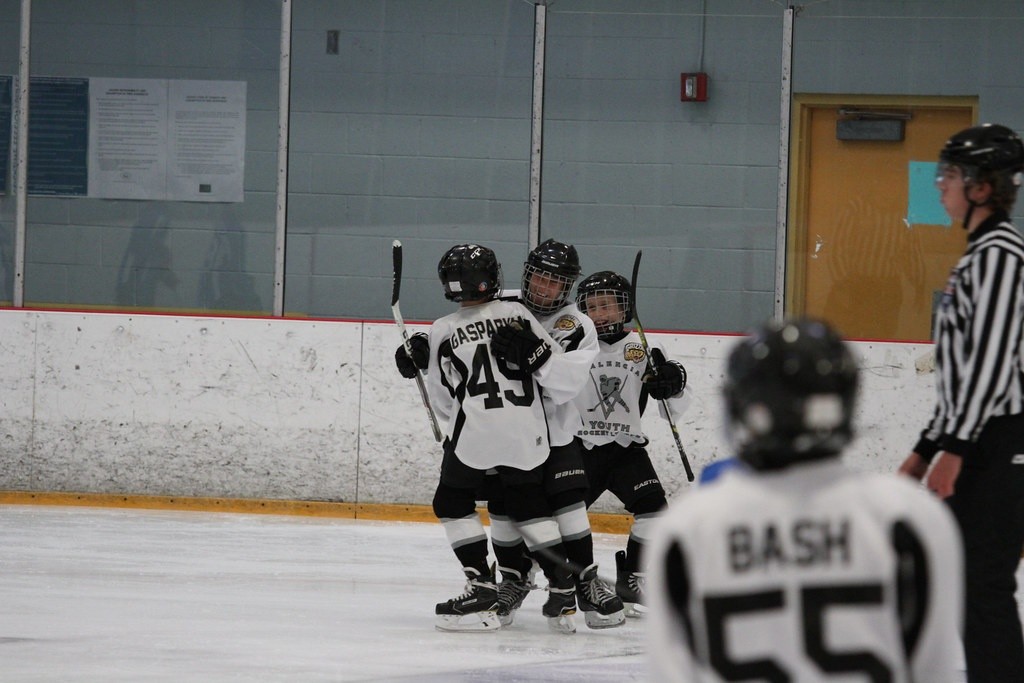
[386,239,444,444]
[631,251,696,483]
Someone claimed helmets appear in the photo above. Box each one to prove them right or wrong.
[520,237,582,317]
[576,271,633,337]
[437,243,504,303]
[721,316,860,472]
[939,123,1024,206]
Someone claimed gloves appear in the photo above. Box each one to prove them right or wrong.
[395,331,430,379]
[489,319,552,373]
[640,347,687,401]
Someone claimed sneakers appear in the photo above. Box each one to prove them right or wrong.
[577,563,626,628]
[496,557,535,625]
[542,574,580,632]
[615,550,651,617]
[434,560,502,634]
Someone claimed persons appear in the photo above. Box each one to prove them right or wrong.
[394,238,694,633]
[640,316,969,683]
[896,122,1024,683]
